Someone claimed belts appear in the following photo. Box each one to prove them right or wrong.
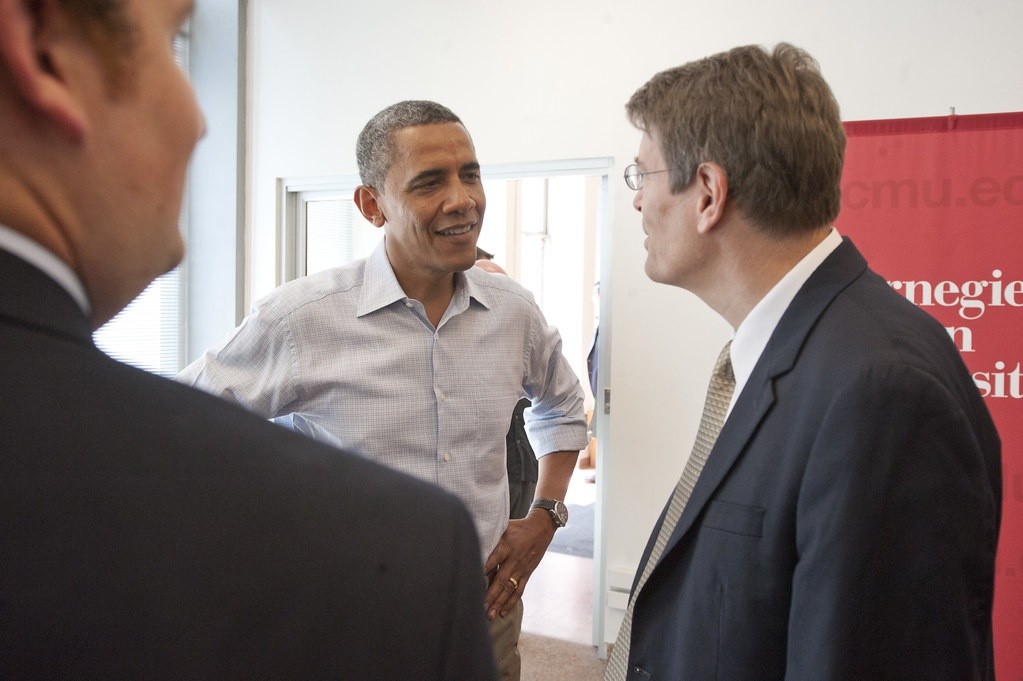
[482,566,498,591]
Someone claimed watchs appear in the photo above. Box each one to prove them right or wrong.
[532,499,568,527]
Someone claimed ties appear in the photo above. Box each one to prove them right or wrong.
[602,341,739,681]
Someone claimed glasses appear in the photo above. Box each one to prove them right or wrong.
[624,163,736,196]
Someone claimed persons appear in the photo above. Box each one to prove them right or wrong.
[587,281,600,484]
[473,248,537,519]
[173,99,587,681]
[607,43,1000,681]
[0,1,496,680]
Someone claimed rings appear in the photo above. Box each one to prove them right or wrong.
[509,578,517,587]
